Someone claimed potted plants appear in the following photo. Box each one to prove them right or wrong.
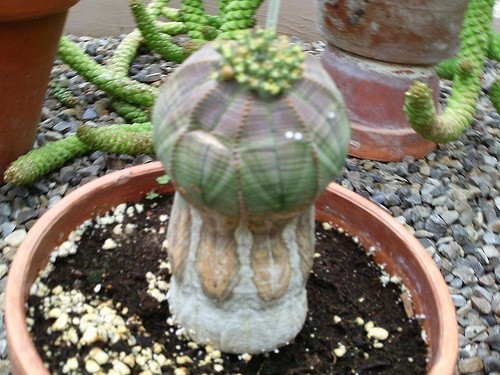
[2,27,458,375]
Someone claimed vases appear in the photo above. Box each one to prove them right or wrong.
[0,0,79,185]
[316,1,472,65]
[316,43,441,162]
